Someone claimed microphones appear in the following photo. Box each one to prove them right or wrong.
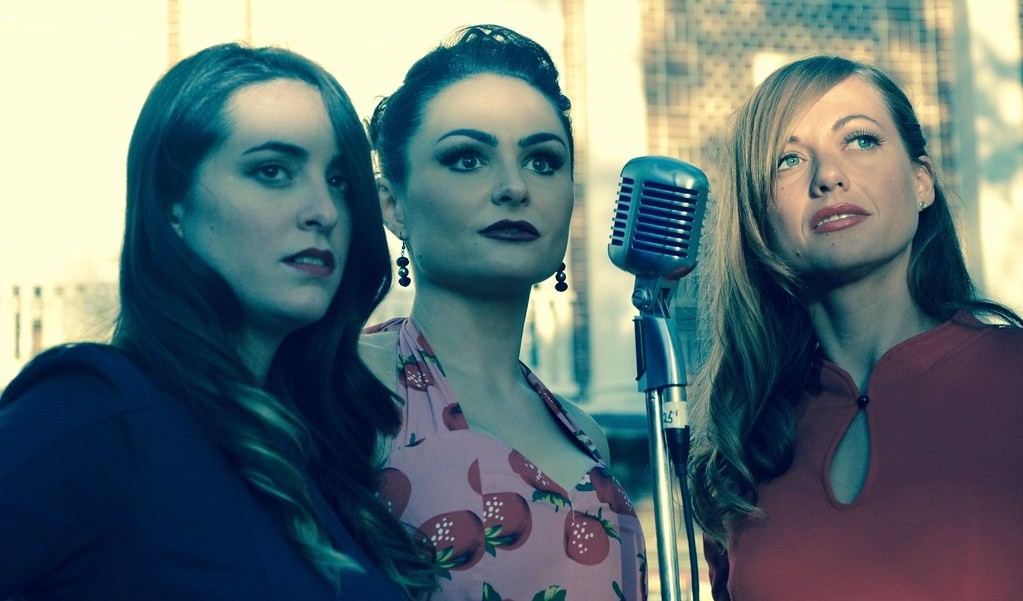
[608,156,711,477]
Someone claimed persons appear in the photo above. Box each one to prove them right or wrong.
[0,40,443,601]
[687,55,1023,601]
[358,23,649,601]
